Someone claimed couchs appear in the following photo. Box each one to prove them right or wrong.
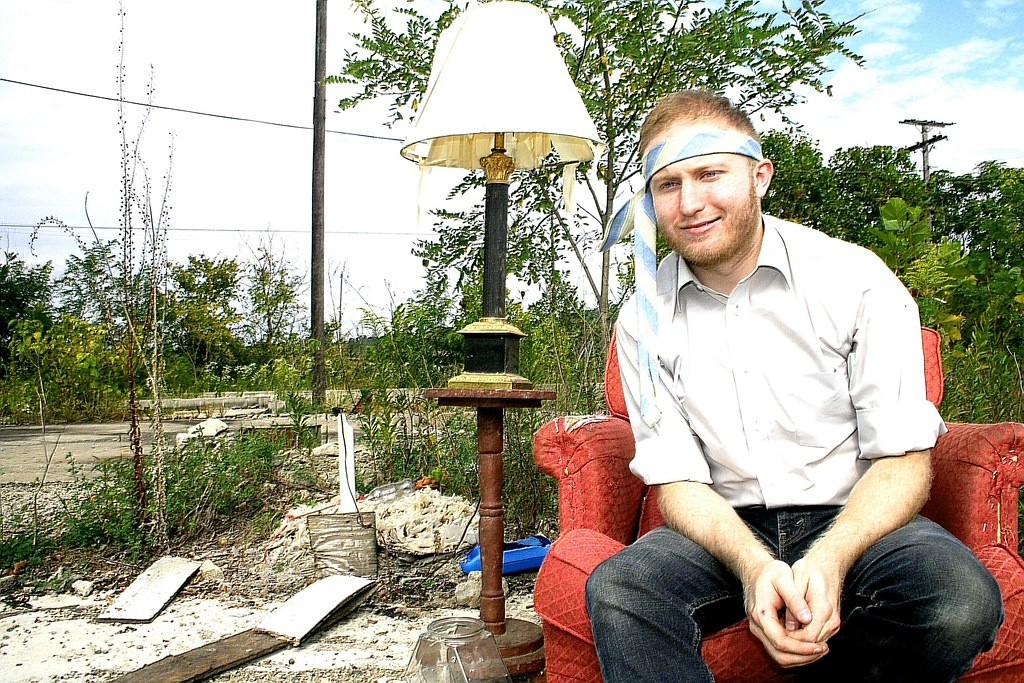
[530,304,1023,682]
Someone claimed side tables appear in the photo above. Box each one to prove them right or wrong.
[415,385,556,681]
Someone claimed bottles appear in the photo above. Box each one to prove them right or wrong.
[402,614,514,683]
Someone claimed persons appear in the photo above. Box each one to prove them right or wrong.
[583,93,1009,683]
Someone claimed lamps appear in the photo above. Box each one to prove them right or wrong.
[398,0,606,394]
